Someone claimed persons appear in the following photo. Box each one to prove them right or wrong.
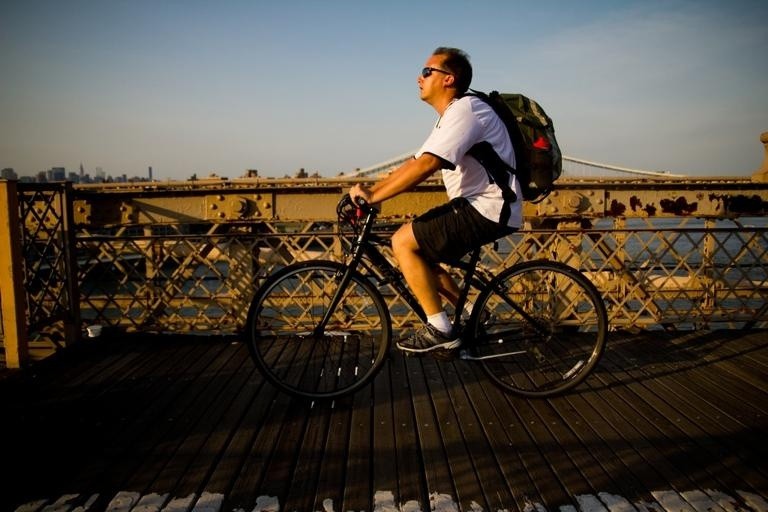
[349,47,524,355]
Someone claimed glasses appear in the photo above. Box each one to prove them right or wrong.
[422,67,448,77]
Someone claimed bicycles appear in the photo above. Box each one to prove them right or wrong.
[245,191,608,400]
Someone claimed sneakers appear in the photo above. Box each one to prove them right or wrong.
[396,309,496,353]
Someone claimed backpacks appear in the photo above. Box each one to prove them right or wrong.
[457,88,562,205]
